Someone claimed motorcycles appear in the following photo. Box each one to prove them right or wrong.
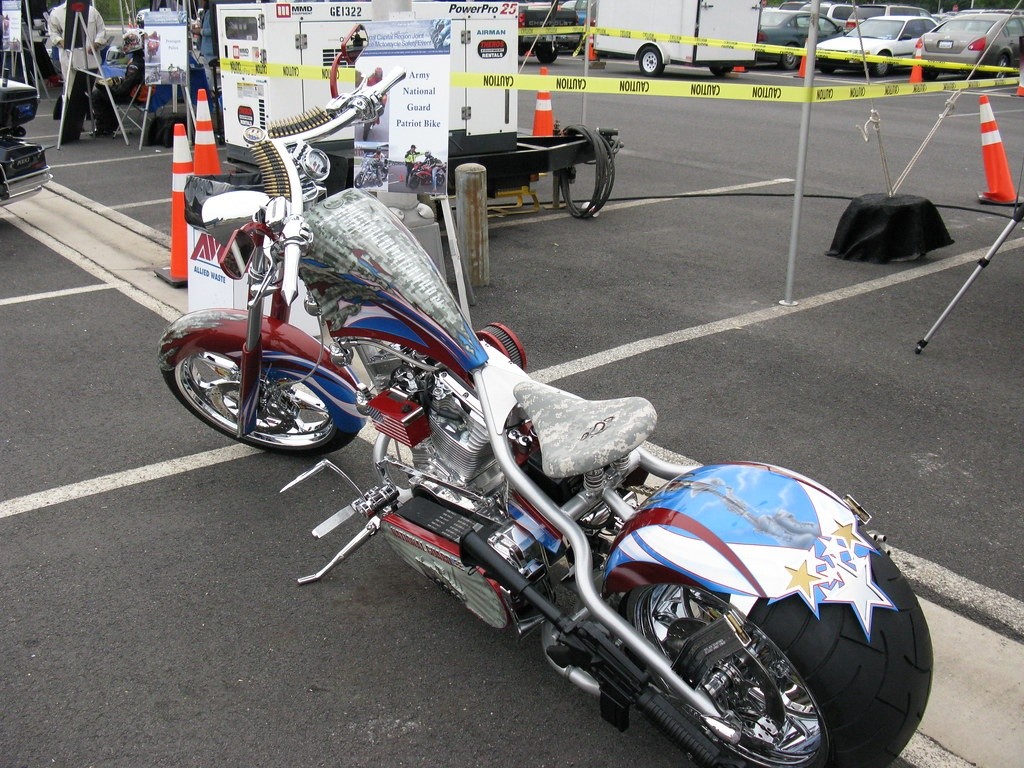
[159,23,935,768]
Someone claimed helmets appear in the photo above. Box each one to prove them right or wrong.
[121,29,148,55]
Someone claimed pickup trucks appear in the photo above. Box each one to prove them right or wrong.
[518,9,583,64]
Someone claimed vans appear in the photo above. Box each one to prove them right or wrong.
[779,2,939,37]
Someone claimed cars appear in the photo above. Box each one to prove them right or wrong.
[920,14,1024,78]
[754,10,847,70]
[816,16,938,76]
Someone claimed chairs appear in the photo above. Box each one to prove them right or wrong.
[92,46,213,138]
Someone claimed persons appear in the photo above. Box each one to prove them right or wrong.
[90,29,156,136]
[373,150,385,187]
[362,68,388,124]
[47,0,107,103]
[136,9,150,29]
[425,152,438,192]
[0,0,65,87]
[191,0,213,55]
[404,145,425,186]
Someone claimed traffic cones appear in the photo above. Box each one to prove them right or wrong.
[532,67,560,136]
[154,123,193,288]
[976,96,1024,205]
[589,33,596,60]
[793,38,808,78]
[910,49,924,82]
[191,89,222,175]
